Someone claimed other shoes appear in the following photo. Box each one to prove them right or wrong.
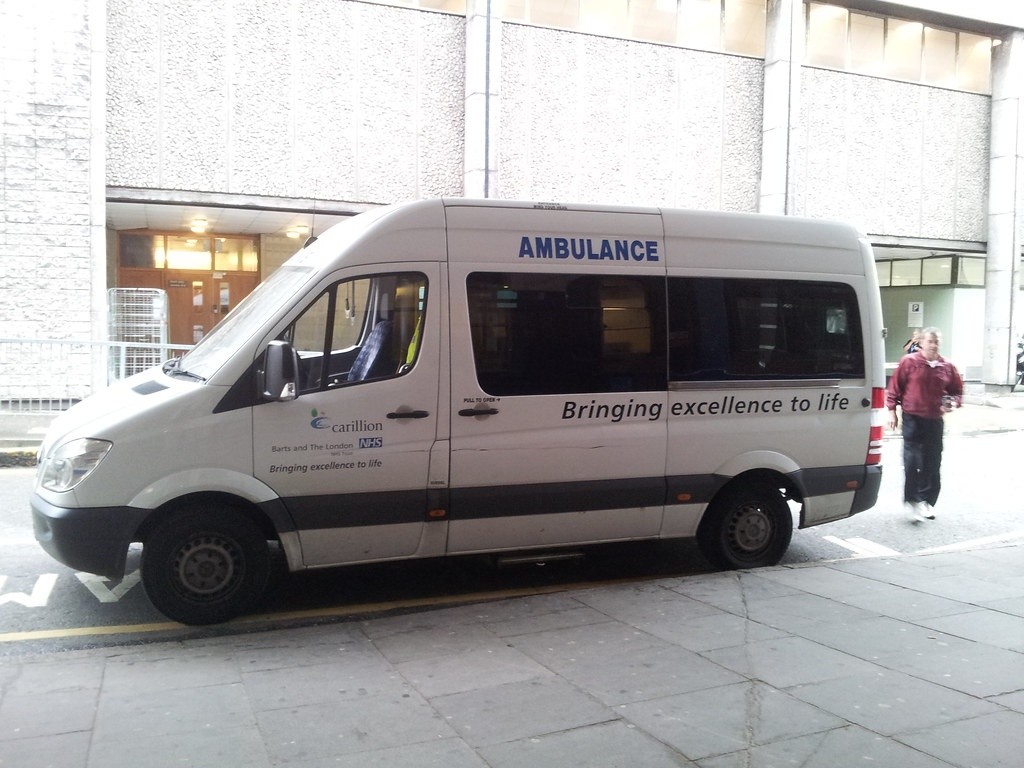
[906,502,923,523]
[916,500,935,520]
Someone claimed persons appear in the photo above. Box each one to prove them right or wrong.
[902,328,923,353]
[886,326,963,525]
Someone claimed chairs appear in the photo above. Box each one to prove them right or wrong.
[349,319,392,381]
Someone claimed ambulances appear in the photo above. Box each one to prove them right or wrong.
[27,196,888,628]
